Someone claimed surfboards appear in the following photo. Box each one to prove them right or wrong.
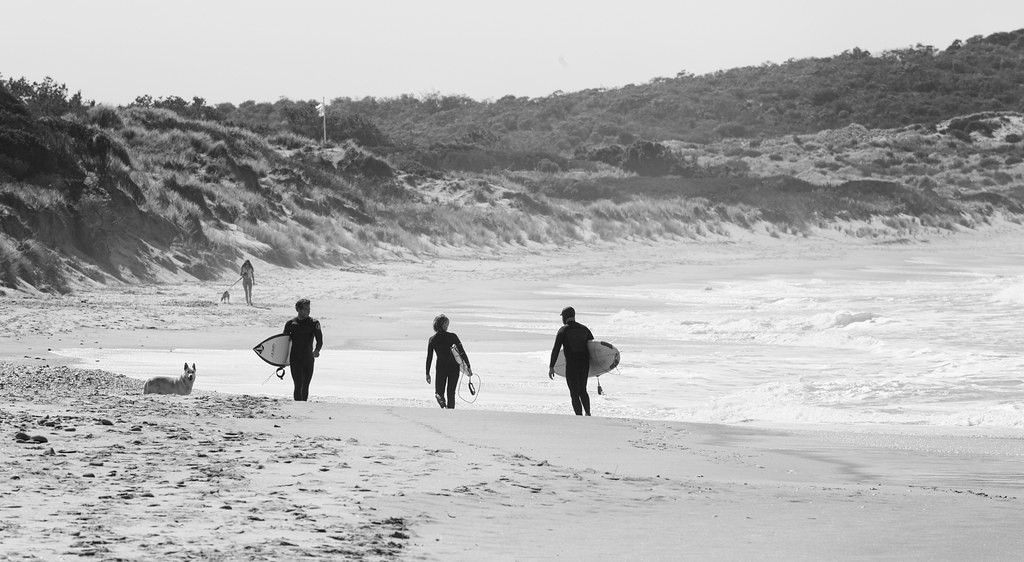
[252,333,293,368]
[551,339,620,377]
[451,345,472,376]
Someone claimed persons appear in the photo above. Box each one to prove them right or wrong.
[549,306,594,416]
[240,259,256,306]
[277,298,324,401]
[425,315,473,410]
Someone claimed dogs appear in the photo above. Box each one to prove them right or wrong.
[143,363,196,395]
[221,291,230,304]
